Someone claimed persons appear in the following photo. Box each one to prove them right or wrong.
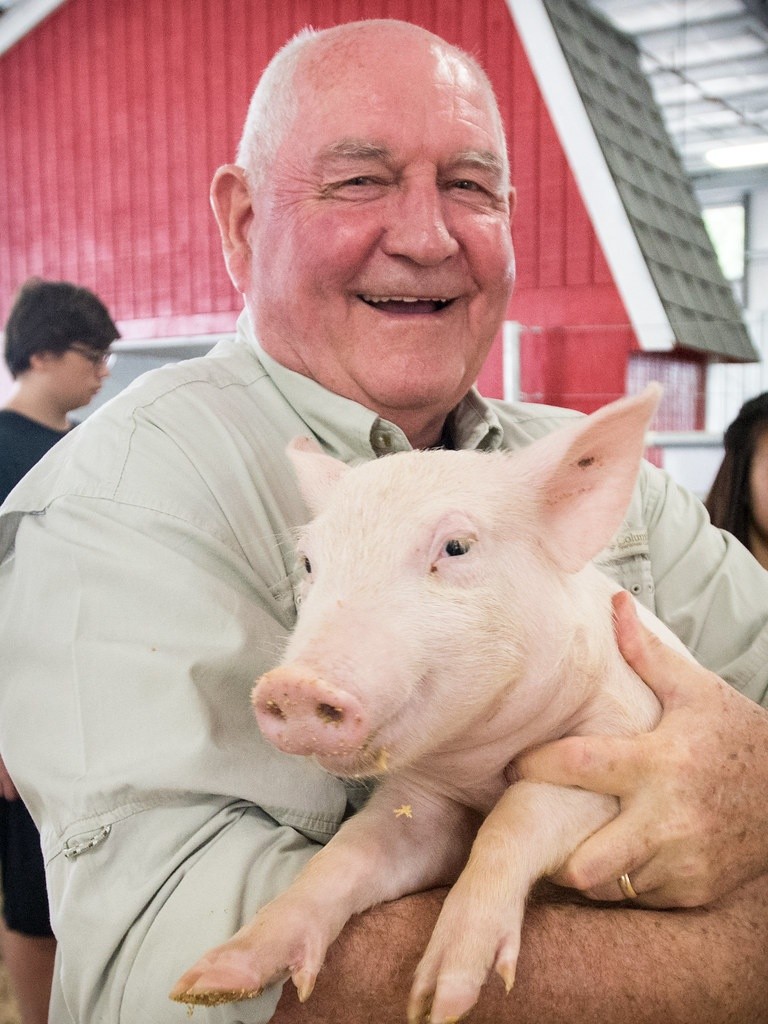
[0,15,764,1024]
[702,394,767,571]
[0,276,124,1024]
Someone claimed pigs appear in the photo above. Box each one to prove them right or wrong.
[169,379,698,1024]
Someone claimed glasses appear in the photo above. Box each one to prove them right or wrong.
[65,345,118,370]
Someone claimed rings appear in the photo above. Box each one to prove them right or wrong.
[618,871,639,901]
